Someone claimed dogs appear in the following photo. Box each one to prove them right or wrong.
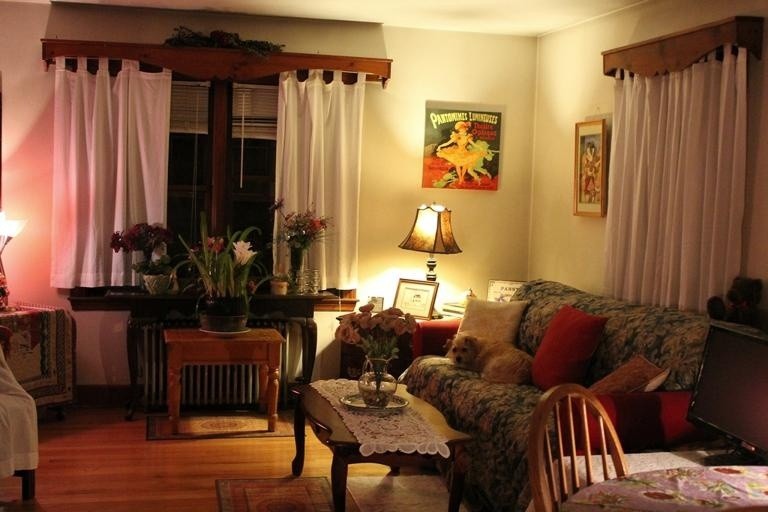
[444,333,536,385]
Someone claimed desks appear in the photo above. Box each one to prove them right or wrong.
[67,286,360,435]
[559,465,768,510]
[335,308,462,380]
[163,328,287,435]
[1,301,76,422]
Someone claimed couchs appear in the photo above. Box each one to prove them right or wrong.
[393,277,711,510]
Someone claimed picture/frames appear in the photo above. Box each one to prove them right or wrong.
[393,277,440,320]
[574,119,608,217]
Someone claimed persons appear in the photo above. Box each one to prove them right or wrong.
[580,142,601,203]
[436,121,480,184]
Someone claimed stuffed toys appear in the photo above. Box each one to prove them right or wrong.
[707,276,763,325]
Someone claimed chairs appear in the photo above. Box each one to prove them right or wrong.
[1,350,39,501]
[526,383,630,511]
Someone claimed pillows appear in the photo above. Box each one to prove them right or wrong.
[586,353,672,395]
[444,294,532,358]
[532,303,612,392]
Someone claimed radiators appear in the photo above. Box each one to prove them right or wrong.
[138,320,294,413]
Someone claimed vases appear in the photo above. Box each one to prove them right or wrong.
[358,354,398,410]
[197,298,249,333]
[291,246,301,285]
[141,272,172,296]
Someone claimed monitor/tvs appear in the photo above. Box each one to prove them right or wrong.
[683,323,768,466]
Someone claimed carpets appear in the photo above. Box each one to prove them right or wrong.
[346,474,469,512]
[147,411,307,441]
[216,476,335,512]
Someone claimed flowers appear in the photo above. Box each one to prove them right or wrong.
[266,195,337,252]
[109,221,179,275]
[335,303,417,391]
[180,211,299,298]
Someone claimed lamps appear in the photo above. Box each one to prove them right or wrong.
[397,201,463,319]
[0,210,27,309]
